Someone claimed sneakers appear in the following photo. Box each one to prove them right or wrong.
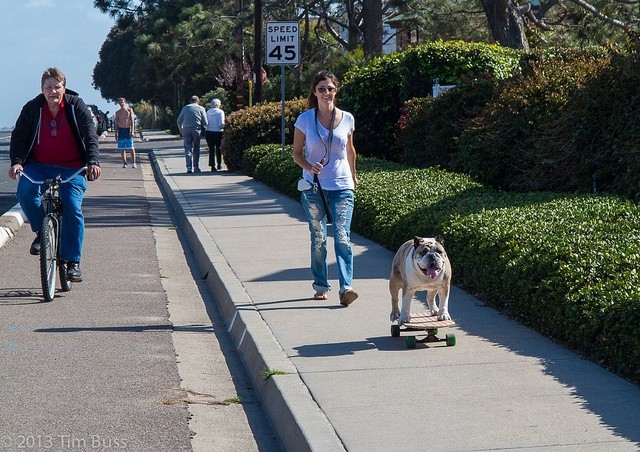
[217,165,222,169]
[123,163,127,168]
[211,167,217,172]
[132,163,137,168]
[194,168,201,173]
[187,169,192,173]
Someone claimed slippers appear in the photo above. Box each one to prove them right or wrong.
[339,290,357,307]
[314,292,328,299]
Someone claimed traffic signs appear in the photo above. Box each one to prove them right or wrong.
[266,21,301,66]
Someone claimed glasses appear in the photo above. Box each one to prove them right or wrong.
[51,120,57,137]
[318,87,336,93]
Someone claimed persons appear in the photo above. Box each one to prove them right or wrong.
[202,99,225,172]
[114,97,138,169]
[8,66,102,283]
[176,95,208,173]
[293,69,359,307]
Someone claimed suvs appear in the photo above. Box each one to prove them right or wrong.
[98,109,106,131]
[87,105,101,136]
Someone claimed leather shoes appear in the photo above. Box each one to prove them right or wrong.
[30,234,40,255]
[67,262,81,281]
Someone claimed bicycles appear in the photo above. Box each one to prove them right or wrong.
[17,166,94,301]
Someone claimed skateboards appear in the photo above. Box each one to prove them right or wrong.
[391,310,456,348]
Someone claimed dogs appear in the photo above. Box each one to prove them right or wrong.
[388,233,451,326]
